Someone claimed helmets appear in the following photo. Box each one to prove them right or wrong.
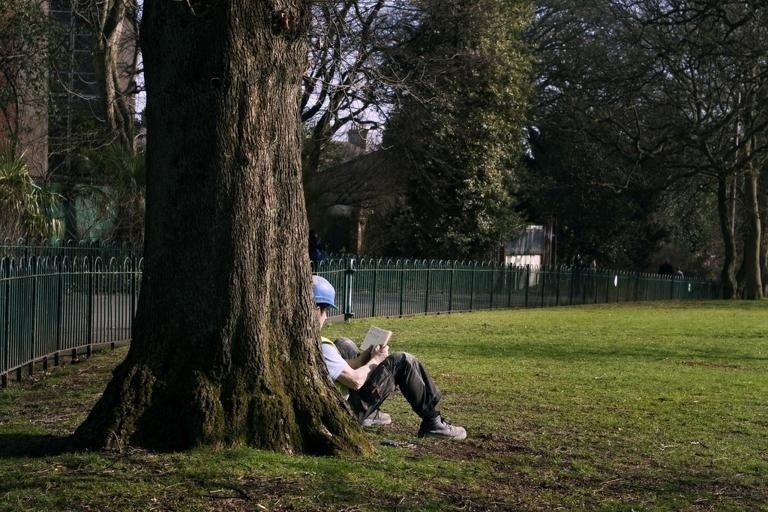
[311,275,339,311]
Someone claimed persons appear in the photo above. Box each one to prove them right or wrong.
[309,230,329,265]
[580,251,598,299]
[674,267,683,276]
[572,254,582,297]
[311,274,467,443]
[657,257,675,277]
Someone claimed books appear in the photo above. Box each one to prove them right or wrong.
[359,323,394,351]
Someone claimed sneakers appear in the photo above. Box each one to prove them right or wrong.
[418,414,467,441]
[362,408,392,427]
